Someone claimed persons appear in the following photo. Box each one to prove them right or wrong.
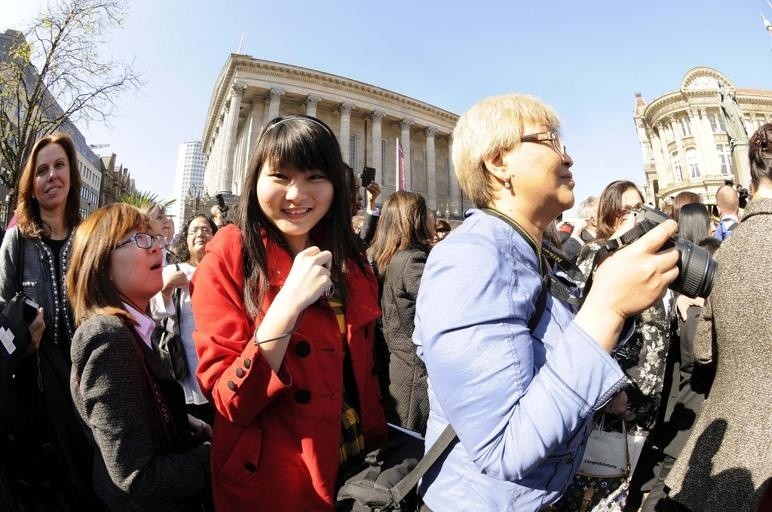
[1,93,772,511]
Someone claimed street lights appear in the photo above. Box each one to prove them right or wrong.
[187,183,212,216]
[4,195,11,228]
[436,200,460,225]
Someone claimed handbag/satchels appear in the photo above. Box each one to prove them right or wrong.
[335,440,422,512]
[557,471,630,512]
[580,429,646,480]
[158,332,188,381]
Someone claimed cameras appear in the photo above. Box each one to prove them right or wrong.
[361,167,376,188]
[635,205,716,300]
[216,194,229,212]
[313,264,335,304]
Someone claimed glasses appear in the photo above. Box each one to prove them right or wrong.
[184,227,212,235]
[109,233,165,249]
[521,130,561,150]
